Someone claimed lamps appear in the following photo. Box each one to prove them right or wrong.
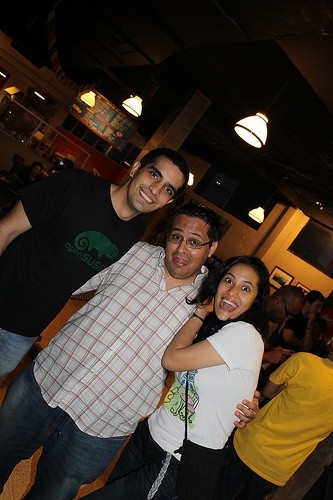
[233,68,297,150]
[248,182,284,224]
[69,91,97,117]
[121,81,154,118]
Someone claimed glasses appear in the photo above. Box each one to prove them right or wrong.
[278,295,296,320]
[167,232,212,249]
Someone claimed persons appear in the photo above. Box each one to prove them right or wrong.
[0,148,273,499]
[213,286,333,500]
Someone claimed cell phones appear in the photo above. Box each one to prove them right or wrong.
[274,347,294,354]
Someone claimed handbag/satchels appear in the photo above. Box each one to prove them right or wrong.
[174,440,228,500]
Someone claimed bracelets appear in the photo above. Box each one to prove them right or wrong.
[192,313,204,322]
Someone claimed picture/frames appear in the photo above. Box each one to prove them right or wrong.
[296,282,310,296]
[267,266,294,291]
